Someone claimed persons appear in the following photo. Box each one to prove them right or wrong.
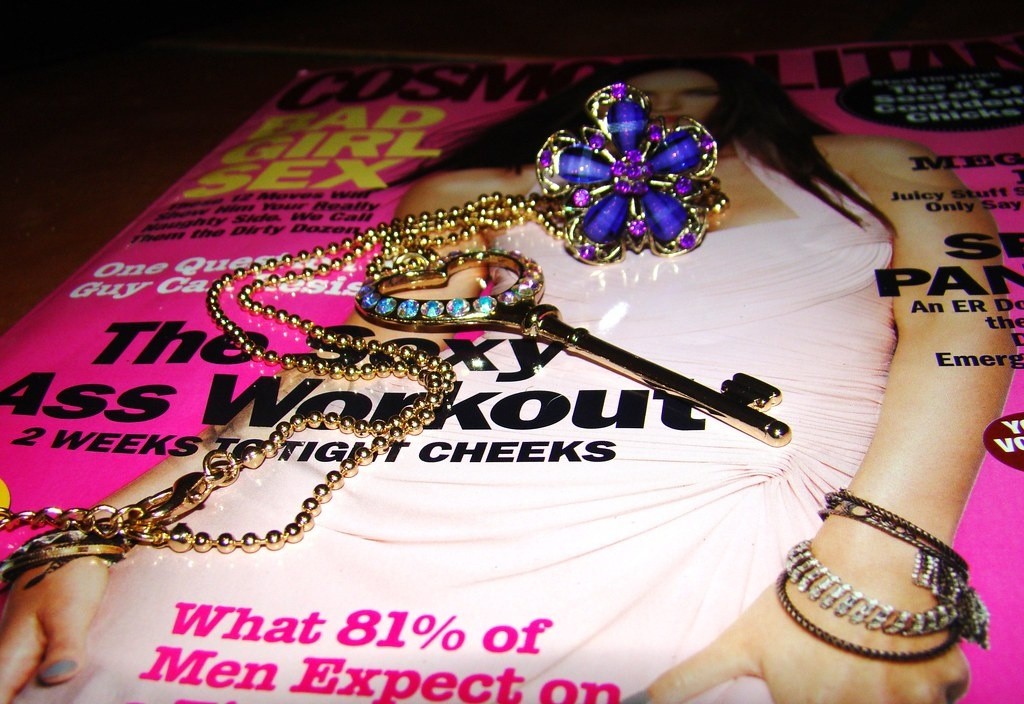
[0,56,1020,704]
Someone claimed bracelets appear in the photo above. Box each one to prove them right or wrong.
[0,526,134,584]
[777,487,990,663]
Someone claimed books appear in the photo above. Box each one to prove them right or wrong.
[0,41,1024,704]
